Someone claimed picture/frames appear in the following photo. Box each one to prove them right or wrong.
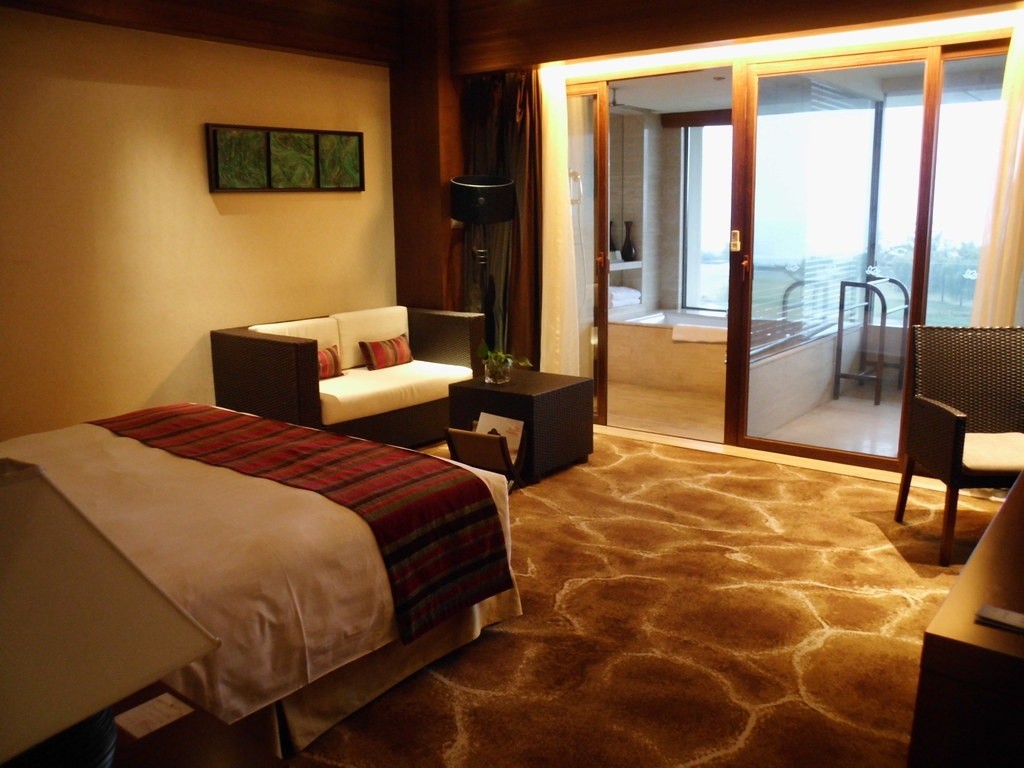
[318,129,365,191]
[205,122,269,193]
[269,127,317,191]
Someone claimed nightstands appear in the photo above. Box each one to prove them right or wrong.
[113,680,286,768]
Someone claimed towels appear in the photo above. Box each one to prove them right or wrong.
[608,299,641,307]
[610,286,641,300]
[672,323,728,344]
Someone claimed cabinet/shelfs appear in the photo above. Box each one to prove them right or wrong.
[449,368,594,481]
[609,261,642,307]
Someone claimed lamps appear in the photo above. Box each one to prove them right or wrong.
[0,455,221,768]
[449,175,517,352]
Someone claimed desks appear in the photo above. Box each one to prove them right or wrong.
[906,471,1024,768]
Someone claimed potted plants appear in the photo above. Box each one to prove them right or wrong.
[482,350,533,384]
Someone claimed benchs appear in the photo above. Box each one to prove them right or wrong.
[894,324,1024,567]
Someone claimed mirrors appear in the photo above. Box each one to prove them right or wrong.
[610,113,623,252]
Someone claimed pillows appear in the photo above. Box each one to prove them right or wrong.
[319,344,344,379]
[359,332,413,371]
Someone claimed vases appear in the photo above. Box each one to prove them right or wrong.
[621,222,637,262]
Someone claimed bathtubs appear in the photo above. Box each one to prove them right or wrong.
[608,309,805,396]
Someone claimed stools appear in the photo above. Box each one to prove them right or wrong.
[444,408,530,494]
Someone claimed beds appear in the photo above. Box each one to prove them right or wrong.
[0,403,522,768]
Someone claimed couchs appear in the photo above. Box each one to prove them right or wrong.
[210,305,484,448]
